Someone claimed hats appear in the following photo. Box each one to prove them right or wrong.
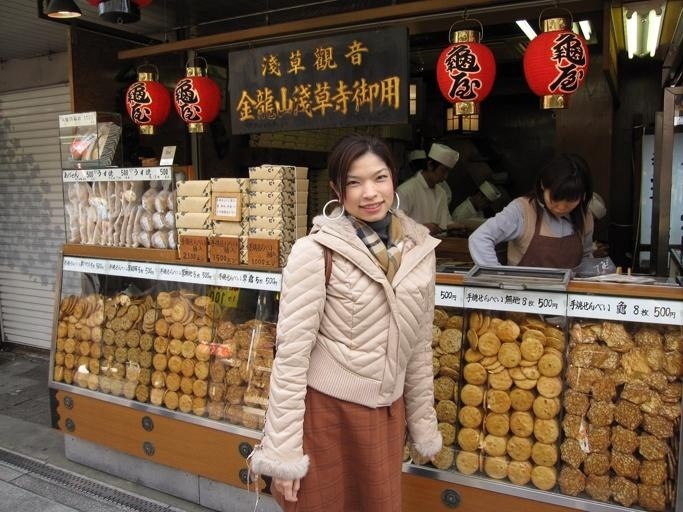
[427,142,461,169]
[406,150,427,163]
[583,190,608,221]
[478,179,503,202]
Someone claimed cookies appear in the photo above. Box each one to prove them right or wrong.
[456,313,565,490]
[52,294,104,390]
[136,180,176,249]
[150,288,221,417]
[98,292,157,402]
[559,321,683,511]
[402,309,465,470]
[207,318,276,430]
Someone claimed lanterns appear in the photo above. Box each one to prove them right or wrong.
[437,30,497,132]
[522,17,590,110]
[173,66,222,133]
[125,71,172,136]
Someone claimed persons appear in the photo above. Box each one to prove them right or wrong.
[587,192,617,258]
[395,150,427,183]
[451,180,503,234]
[391,143,470,238]
[246,129,444,512]
[466,153,597,278]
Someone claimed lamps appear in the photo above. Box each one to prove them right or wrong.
[48,0,81,20]
[621,0,668,60]
[579,19,594,41]
[571,21,579,34]
[514,17,538,41]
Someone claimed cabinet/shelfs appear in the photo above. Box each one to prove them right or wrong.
[47,244,683,512]
